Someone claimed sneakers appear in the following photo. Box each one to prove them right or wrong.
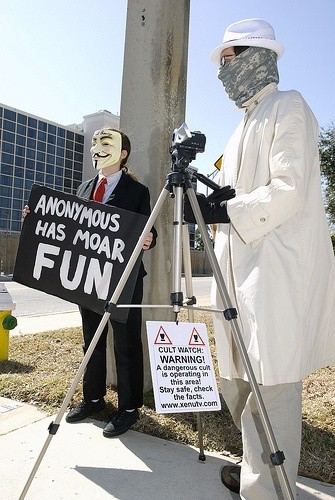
[220,465,242,495]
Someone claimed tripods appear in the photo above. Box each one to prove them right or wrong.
[19,146,294,500]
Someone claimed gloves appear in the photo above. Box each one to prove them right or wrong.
[206,185,235,204]
[184,192,230,224]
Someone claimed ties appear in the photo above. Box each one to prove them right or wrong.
[92,177,107,202]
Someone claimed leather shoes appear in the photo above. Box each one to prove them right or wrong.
[66,399,106,423]
[103,408,139,438]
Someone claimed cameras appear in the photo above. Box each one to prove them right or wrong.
[171,131,207,153]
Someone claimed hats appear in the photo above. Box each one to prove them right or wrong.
[210,18,285,64]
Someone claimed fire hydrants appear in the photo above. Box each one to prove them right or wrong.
[0,283,18,361]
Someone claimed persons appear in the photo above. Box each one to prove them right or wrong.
[184,19,335,500]
[22,130,158,435]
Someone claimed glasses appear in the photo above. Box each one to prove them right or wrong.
[221,54,236,65]
